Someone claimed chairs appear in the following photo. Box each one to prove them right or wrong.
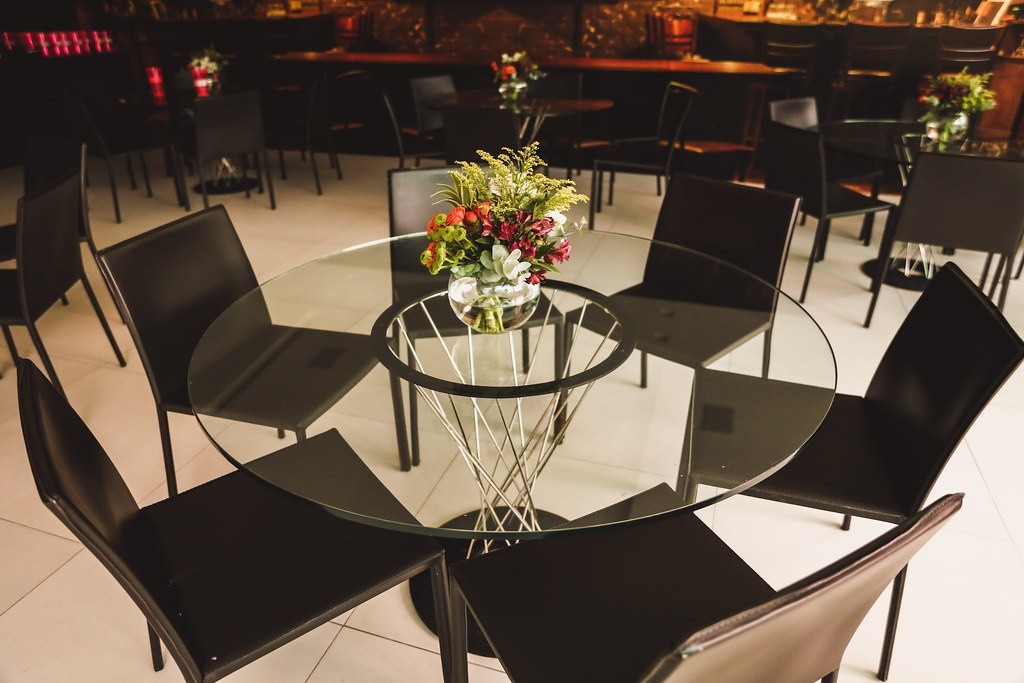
[0,9,1024,683]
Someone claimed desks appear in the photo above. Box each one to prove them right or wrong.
[268,49,807,196]
[826,120,1024,292]
[186,228,838,558]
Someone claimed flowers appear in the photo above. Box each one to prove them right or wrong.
[917,65,998,131]
[422,142,590,296]
[191,55,219,89]
[492,52,547,83]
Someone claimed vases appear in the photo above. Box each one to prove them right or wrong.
[448,273,543,336]
[498,81,530,101]
[927,113,969,142]
